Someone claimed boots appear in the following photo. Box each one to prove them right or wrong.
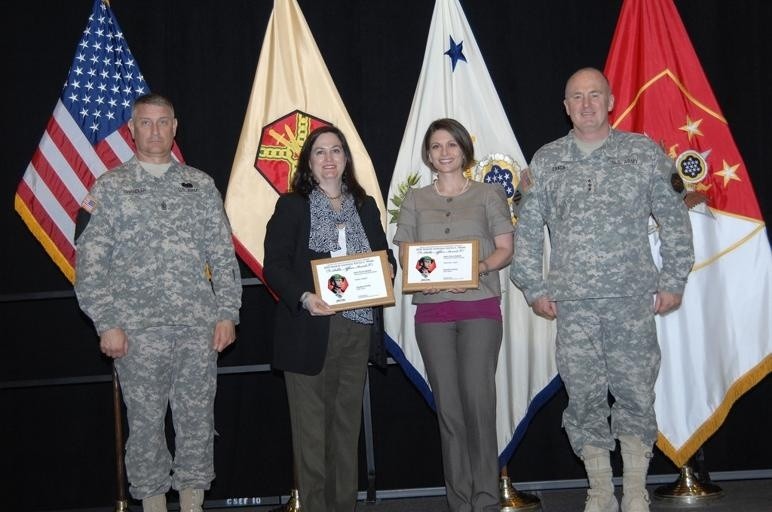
[178,487,204,512]
[582,443,619,512]
[143,494,168,512]
[618,435,655,512]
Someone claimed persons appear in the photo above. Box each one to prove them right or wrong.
[74,93,243,512]
[392,118,516,512]
[509,67,696,512]
[418,255,435,273]
[329,274,346,294]
[261,126,398,512]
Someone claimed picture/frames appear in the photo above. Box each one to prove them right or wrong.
[400,238,481,294]
[310,248,398,311]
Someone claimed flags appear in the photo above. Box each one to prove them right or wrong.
[379,0,557,470]
[15,0,186,286]
[604,0,772,469]
[222,0,386,302]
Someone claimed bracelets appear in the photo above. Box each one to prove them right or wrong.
[478,261,488,272]
